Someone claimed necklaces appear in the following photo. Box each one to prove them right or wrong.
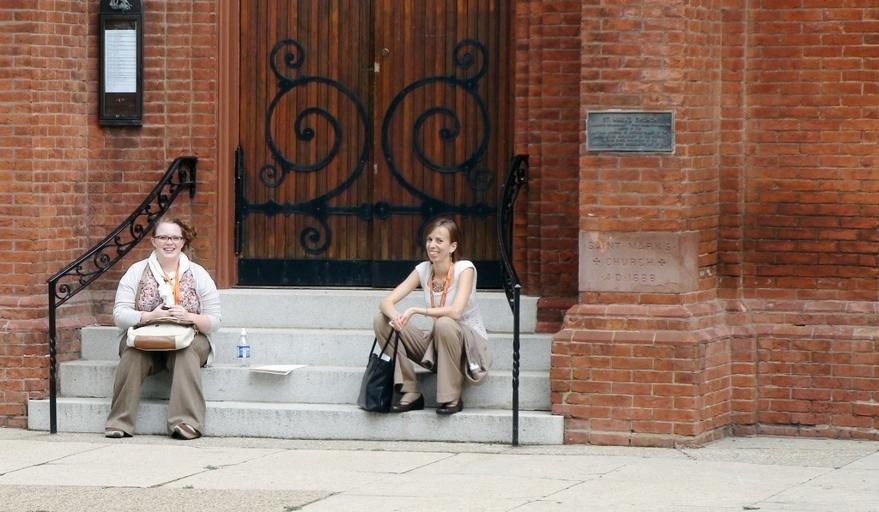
[431,281,445,292]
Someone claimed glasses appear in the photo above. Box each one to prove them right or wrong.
[153,235,184,242]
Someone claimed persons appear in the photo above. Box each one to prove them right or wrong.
[373,219,494,415]
[104,216,221,440]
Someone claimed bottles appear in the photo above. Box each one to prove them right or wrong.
[236,328,250,368]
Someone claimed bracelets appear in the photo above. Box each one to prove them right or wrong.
[424,307,429,318]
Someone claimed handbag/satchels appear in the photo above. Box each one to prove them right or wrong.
[355,324,401,414]
[125,319,195,353]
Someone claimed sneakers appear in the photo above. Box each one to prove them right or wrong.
[103,427,125,438]
[169,421,203,440]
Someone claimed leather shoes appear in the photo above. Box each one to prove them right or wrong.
[434,399,464,414]
[391,390,425,412]
